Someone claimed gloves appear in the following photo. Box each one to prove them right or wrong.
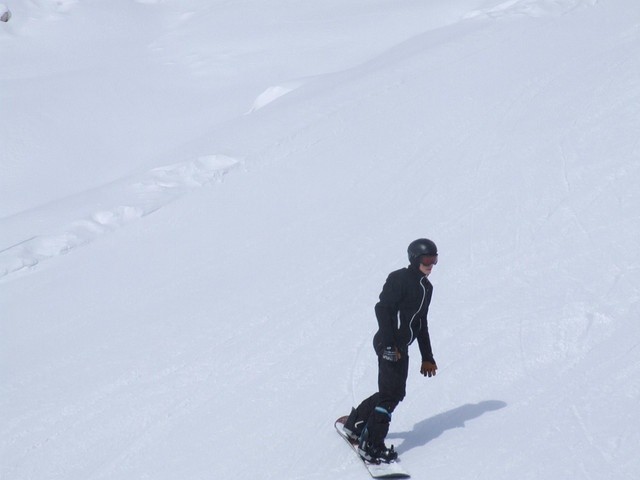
[421,359,437,377]
[383,346,401,362]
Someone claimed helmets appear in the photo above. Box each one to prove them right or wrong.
[408,239,437,263]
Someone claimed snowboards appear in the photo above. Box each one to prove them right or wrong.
[334,413,411,480]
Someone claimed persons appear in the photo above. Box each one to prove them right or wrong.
[343,238,439,463]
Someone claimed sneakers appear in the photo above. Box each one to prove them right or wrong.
[358,441,398,465]
[343,407,367,442]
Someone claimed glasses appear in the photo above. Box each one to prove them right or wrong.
[414,254,438,266]
